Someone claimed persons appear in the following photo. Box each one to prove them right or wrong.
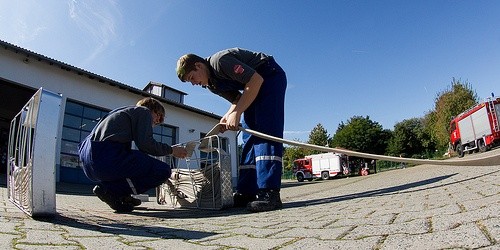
[77,96,188,214]
[178,47,288,213]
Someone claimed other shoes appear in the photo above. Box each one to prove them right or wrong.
[92,182,141,213]
[246,189,282,210]
[220,192,257,210]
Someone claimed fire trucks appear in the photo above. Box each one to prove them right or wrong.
[448,97,500,158]
[292,152,349,182]
